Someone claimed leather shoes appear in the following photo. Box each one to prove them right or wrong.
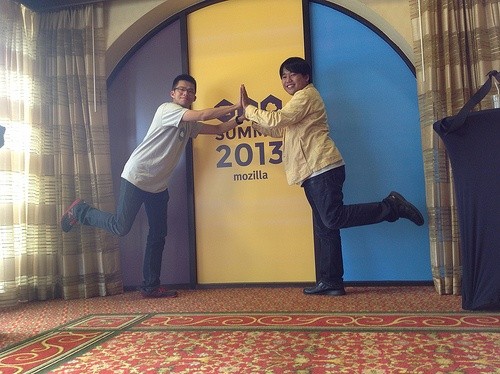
[389,190,424,226]
[303,281,346,295]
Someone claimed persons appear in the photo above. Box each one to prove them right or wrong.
[241,56,425,296]
[60,74,242,300]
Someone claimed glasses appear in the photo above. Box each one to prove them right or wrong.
[173,87,195,95]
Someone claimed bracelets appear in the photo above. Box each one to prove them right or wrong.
[235,115,244,125]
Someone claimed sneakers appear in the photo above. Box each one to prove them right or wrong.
[141,289,178,298]
[61,199,84,232]
